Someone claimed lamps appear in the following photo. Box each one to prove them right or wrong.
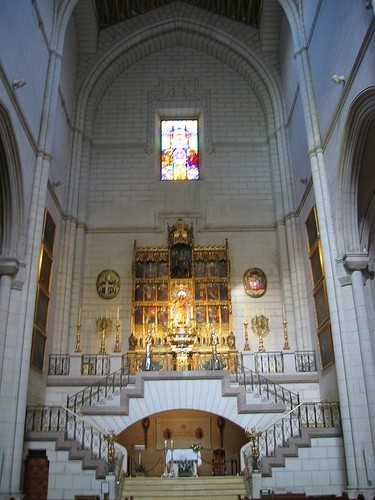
[108,434,116,472]
[142,418,150,449]
[216,416,226,447]
[252,431,261,470]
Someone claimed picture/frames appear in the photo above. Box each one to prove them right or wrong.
[30,208,57,375]
[304,206,335,370]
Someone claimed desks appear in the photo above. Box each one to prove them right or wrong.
[165,448,202,477]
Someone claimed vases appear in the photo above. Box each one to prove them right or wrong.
[194,451,198,460]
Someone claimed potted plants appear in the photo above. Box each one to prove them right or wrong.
[135,464,146,477]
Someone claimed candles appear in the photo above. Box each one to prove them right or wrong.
[283,303,286,321]
[249,302,270,320]
[165,440,167,448]
[96,308,113,321]
[245,303,248,323]
[171,440,174,448]
[78,307,81,326]
[116,307,120,322]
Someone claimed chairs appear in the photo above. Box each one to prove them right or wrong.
[211,448,226,476]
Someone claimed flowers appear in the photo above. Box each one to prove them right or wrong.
[191,442,203,450]
